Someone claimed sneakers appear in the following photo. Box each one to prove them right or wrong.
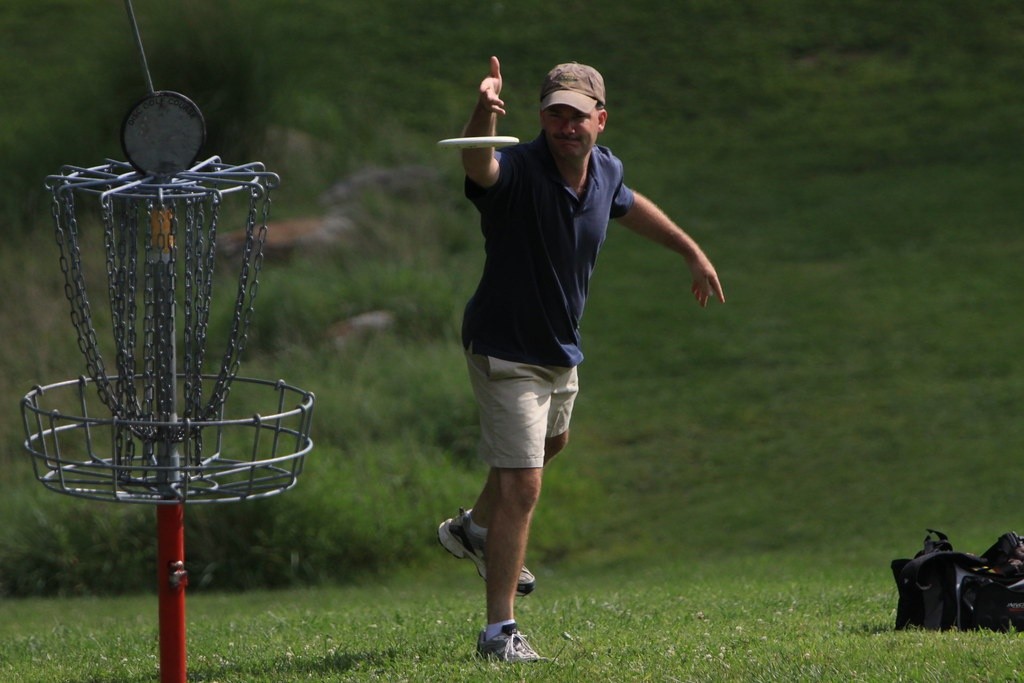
[476,621,550,664]
[437,507,535,597]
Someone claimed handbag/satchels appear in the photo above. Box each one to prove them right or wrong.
[891,528,1024,630]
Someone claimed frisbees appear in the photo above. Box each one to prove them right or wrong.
[437,136,520,149]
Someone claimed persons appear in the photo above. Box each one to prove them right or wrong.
[437,56,725,665]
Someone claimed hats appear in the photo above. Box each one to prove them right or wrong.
[541,60,606,113]
